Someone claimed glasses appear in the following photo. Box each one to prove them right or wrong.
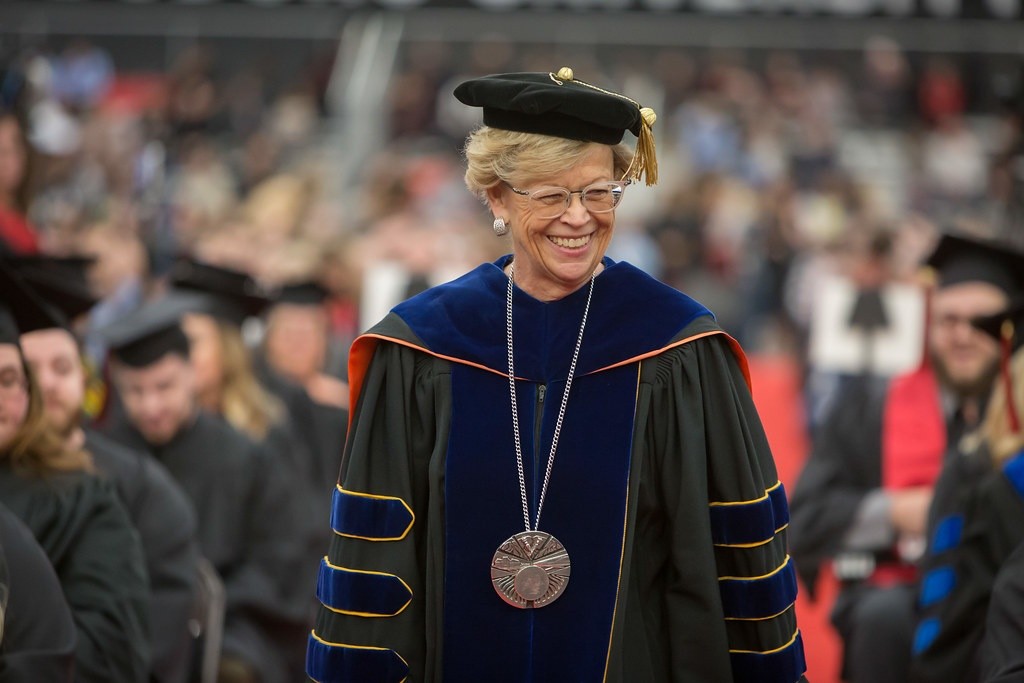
[504,168,632,220]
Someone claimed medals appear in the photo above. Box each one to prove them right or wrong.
[491,531,570,608]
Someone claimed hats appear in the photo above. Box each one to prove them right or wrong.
[0,71,34,124]
[924,233,1024,301]
[0,241,342,368]
[454,67,657,186]
[972,306,1023,429]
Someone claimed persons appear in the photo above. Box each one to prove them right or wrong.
[626,20,1024,683]
[307,67,809,683]
[0,43,490,683]
